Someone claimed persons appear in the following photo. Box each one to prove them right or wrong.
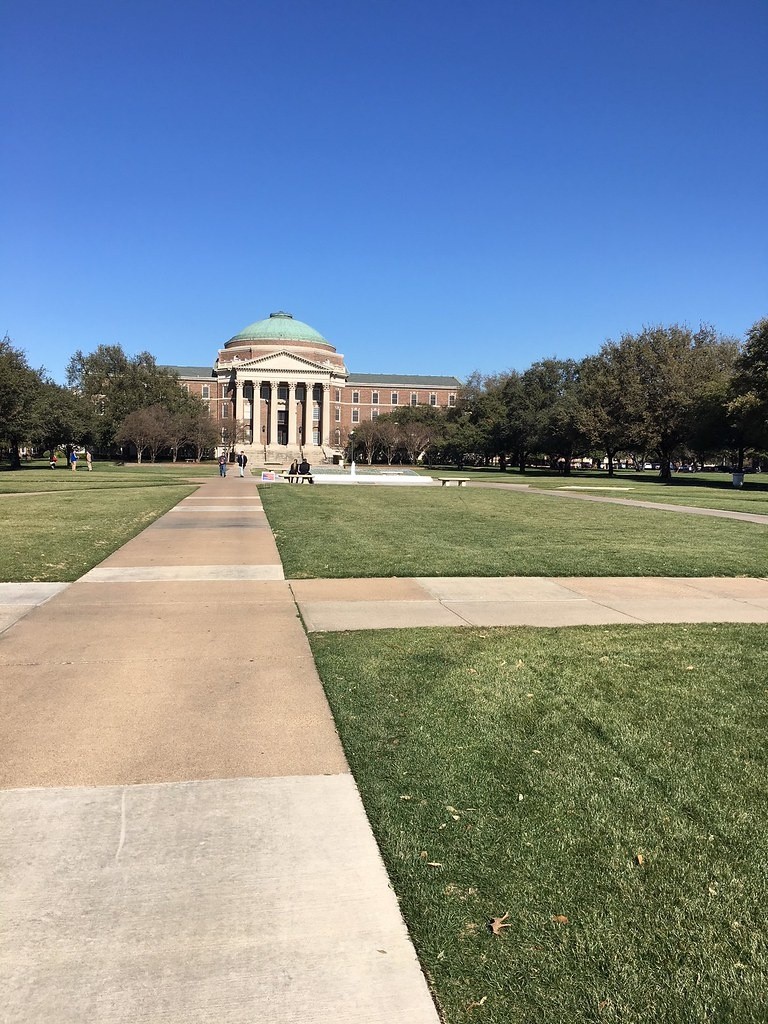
[298,458,312,483]
[86,448,92,471]
[50,454,58,470]
[287,459,298,483]
[218,452,228,477]
[237,450,247,477]
[70,448,79,471]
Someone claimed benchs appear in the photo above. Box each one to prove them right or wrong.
[438,477,470,486]
[279,474,314,483]
[185,459,195,462]
